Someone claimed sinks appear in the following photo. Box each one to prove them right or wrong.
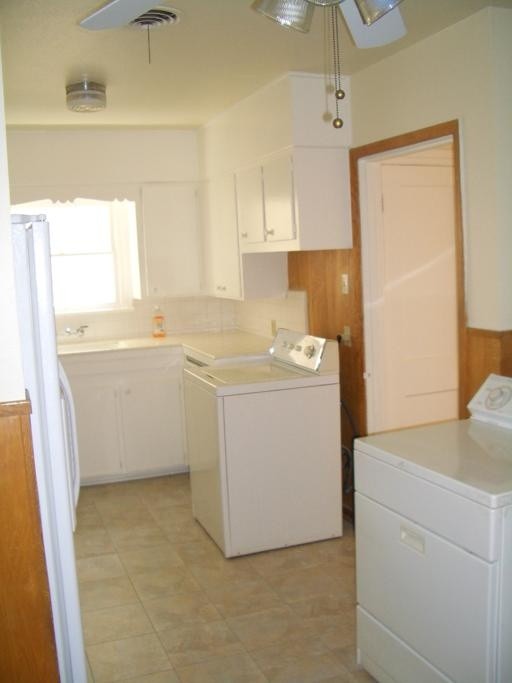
[57,339,128,353]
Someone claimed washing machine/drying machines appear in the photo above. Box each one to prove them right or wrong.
[352,373,512,682]
[182,328,344,559]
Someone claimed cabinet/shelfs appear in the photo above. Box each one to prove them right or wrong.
[202,147,354,302]
[125,174,206,302]
[56,346,188,487]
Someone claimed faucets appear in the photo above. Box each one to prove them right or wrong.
[63,324,88,337]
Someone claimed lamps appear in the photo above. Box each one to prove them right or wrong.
[64,81,109,114]
[259,1,407,128]
